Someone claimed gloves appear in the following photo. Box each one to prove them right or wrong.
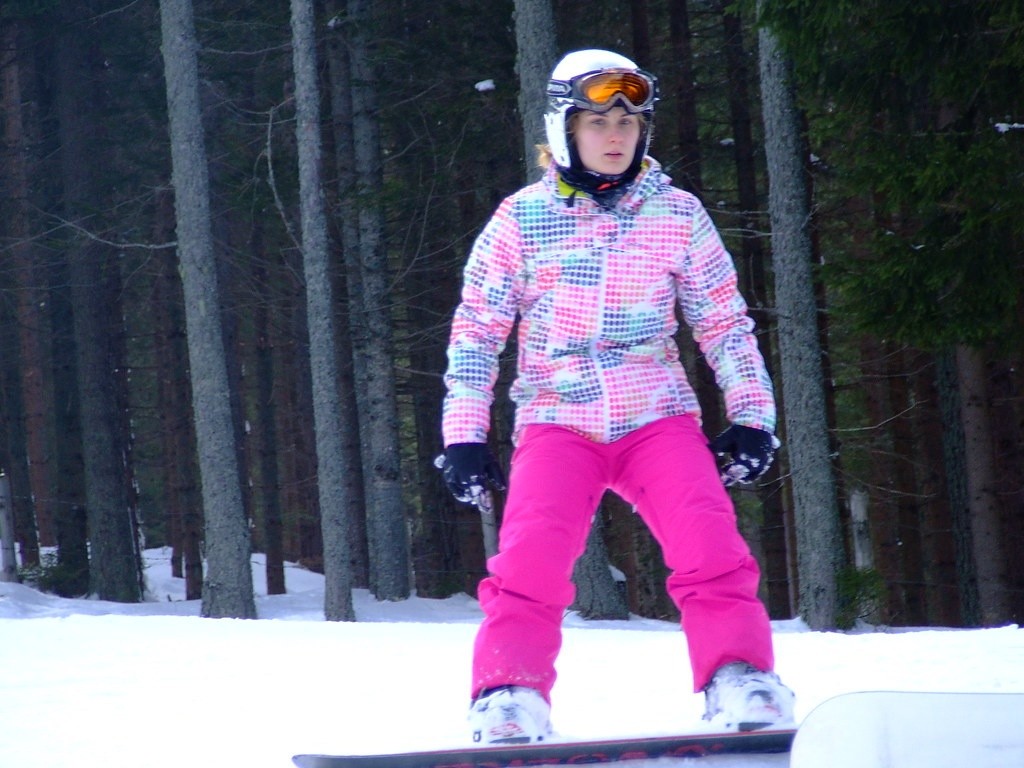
[442,442,506,514]
[705,425,775,488]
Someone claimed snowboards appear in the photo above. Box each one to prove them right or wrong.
[789,692,1024,768]
[291,727,801,768]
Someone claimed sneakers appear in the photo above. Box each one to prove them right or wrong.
[704,661,795,730]
[471,686,553,746]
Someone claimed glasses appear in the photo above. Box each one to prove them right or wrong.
[573,68,660,113]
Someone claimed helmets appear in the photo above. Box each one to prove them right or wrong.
[544,49,652,195]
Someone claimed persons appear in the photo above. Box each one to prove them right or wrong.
[441,51,792,746]
